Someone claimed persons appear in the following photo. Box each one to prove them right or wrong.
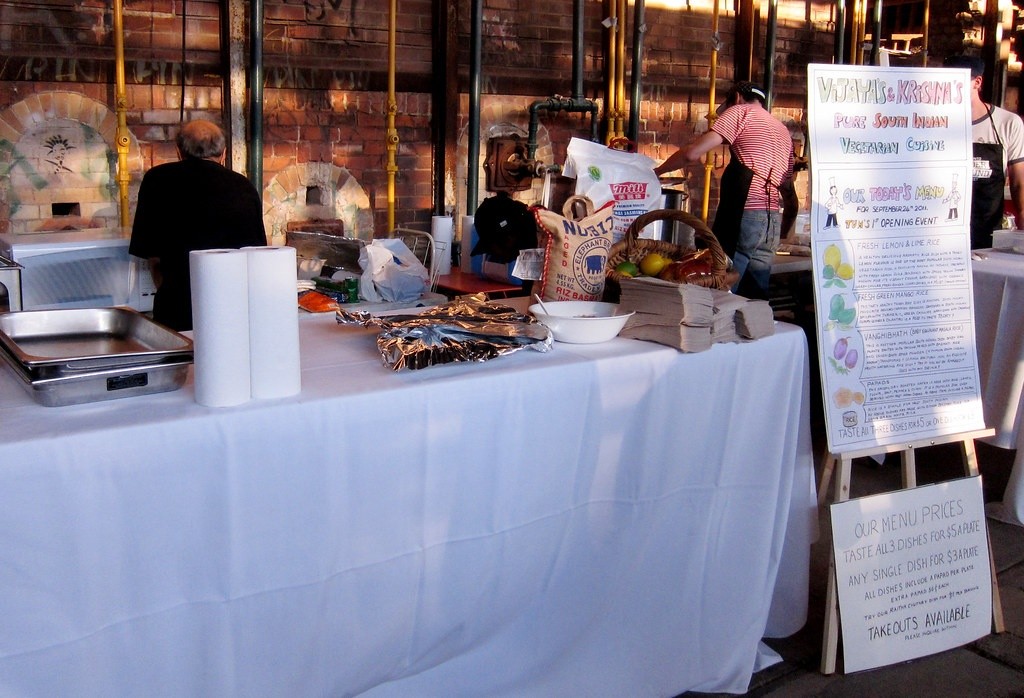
[653,82,794,309]
[130,120,269,333]
[941,56,1023,251]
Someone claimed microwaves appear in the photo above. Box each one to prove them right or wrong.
[0,227,158,312]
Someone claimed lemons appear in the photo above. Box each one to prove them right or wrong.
[615,254,673,278]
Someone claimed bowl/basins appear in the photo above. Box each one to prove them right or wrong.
[528,300,637,345]
[991,230,1024,254]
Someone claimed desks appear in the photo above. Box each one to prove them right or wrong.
[0,248,1024,698]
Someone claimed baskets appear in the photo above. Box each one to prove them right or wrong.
[605,208,739,292]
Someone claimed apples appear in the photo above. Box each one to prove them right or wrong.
[658,247,715,281]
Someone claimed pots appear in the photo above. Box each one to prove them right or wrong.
[655,188,689,245]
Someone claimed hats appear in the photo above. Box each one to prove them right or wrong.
[716,81,767,117]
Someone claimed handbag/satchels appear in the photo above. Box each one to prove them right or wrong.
[358,239,431,303]
[533,195,616,302]
